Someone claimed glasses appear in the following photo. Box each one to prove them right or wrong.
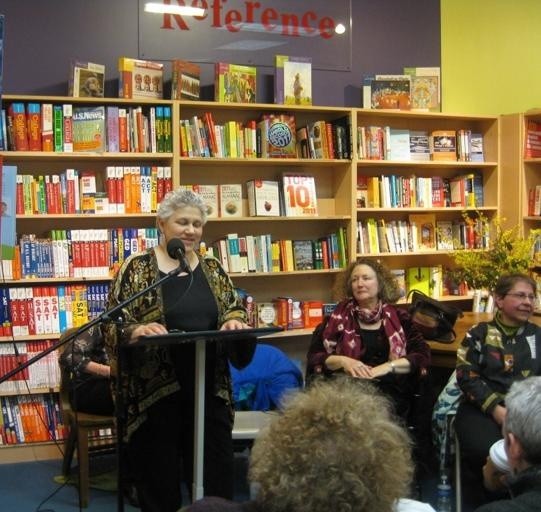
[508,292,535,303]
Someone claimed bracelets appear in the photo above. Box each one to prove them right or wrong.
[390,361,395,373]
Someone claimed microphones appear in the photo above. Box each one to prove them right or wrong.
[167,237,192,274]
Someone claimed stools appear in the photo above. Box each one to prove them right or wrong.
[230,409,279,440]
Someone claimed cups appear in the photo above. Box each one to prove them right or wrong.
[482,436,516,492]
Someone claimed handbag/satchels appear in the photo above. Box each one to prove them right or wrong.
[408,292,464,343]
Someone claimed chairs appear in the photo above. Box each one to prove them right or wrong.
[58,327,120,512]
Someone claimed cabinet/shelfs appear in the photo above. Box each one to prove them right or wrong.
[500,112,541,250]
[354,110,502,311]
[177,101,358,379]
[1,95,178,463]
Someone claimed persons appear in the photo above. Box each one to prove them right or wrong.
[308,257,431,425]
[101,187,255,512]
[181,377,417,512]
[451,271,541,512]
[61,320,114,416]
[474,376,541,512]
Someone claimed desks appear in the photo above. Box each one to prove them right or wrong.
[424,311,541,372]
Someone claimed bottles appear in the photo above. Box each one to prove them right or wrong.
[472,294,487,314]
[435,473,454,512]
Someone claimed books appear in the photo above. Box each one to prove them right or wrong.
[1,340,61,393]
[361,74,412,112]
[402,66,441,113]
[528,185,541,216]
[66,57,106,98]
[181,172,318,217]
[2,100,174,282]
[528,236,541,264]
[357,173,484,207]
[389,264,489,301]
[273,54,313,106]
[178,112,353,160]
[117,56,164,99]
[358,213,490,253]
[214,61,257,104]
[524,119,541,159]
[357,125,484,162]
[170,58,201,101]
[246,295,337,331]
[200,226,349,273]
[0,393,113,448]
[0,281,113,339]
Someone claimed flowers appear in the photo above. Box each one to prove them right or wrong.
[433,197,541,295]
